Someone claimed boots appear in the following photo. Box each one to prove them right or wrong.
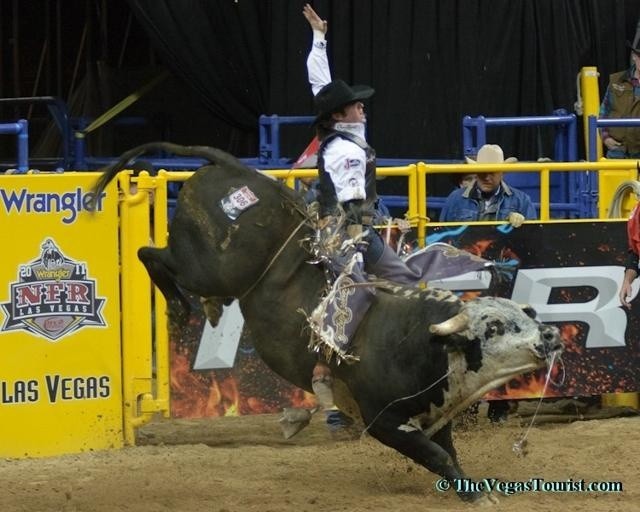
[311,370,353,430]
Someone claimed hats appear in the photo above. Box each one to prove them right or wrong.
[464,143,518,164]
[624,18,640,57]
[306,81,375,129]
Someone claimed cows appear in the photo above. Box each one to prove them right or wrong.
[81,141,565,508]
